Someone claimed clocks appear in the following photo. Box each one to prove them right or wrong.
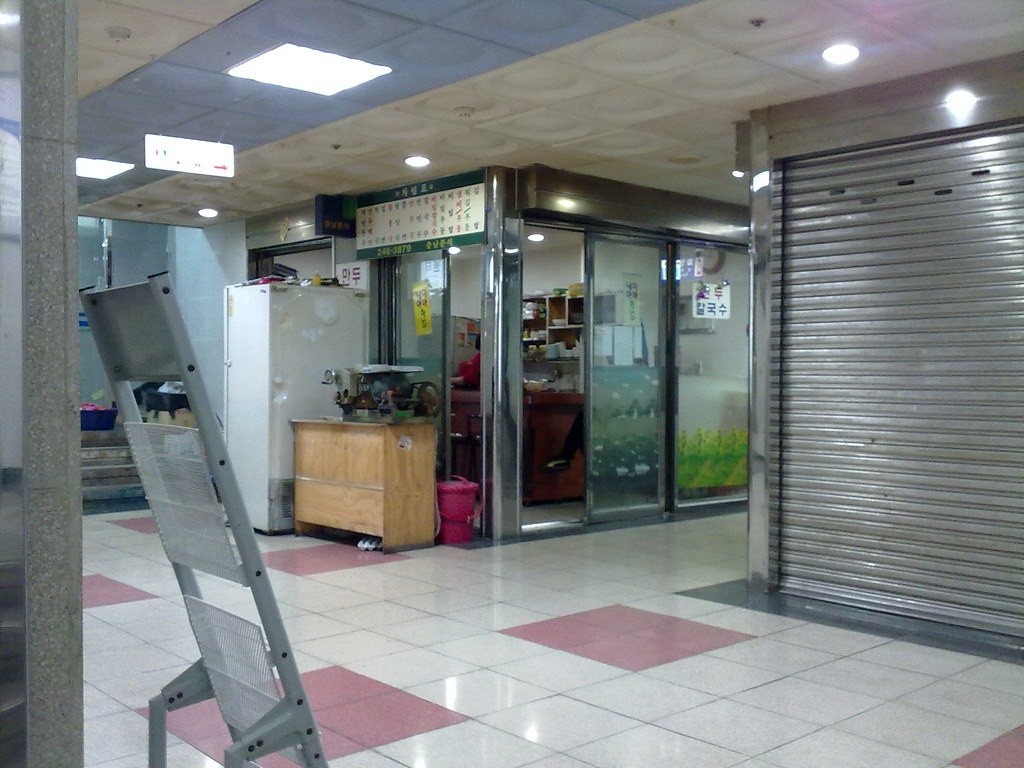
[696,248,725,274]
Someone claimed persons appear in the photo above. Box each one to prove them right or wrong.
[537,410,584,470]
[450,334,481,385]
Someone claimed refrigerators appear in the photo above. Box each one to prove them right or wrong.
[224,278,369,533]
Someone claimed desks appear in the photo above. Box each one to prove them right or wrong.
[290,416,440,555]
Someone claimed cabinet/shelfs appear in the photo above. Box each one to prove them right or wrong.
[521,292,639,392]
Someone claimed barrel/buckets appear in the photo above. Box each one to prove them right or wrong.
[437,475,484,545]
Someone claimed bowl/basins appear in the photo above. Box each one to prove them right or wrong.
[81,407,117,430]
[523,381,545,393]
[552,319,565,326]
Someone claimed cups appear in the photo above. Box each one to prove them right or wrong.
[573,347,578,357]
[565,349,572,357]
[548,344,558,358]
[556,342,566,357]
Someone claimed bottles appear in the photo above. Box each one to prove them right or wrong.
[313,270,321,287]
[524,328,529,338]
